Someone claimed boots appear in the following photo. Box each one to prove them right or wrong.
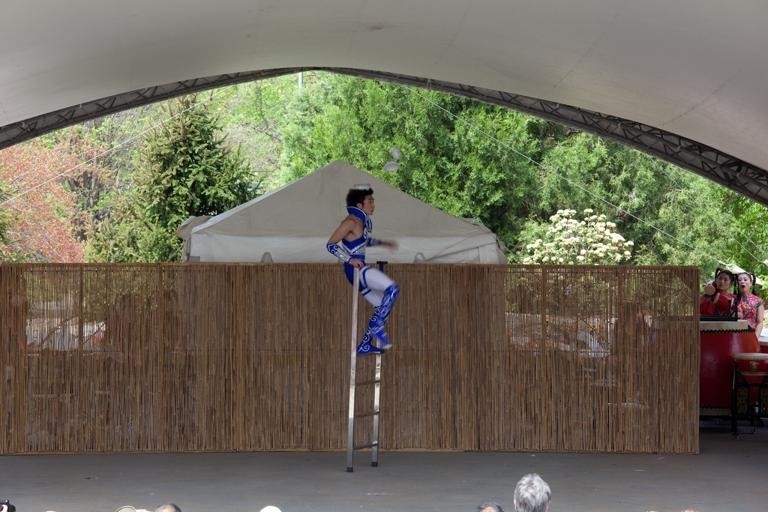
[357,306,384,354]
[372,285,400,344]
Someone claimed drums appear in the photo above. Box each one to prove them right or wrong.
[700,321,762,417]
[736,352,767,383]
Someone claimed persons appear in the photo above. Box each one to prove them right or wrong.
[729,271,764,340]
[513,472,552,512]
[698,267,734,318]
[0,499,282,512]
[610,303,651,375]
[477,503,504,512]
[327,185,401,355]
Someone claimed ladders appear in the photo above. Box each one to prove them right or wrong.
[346,261,388,472]
